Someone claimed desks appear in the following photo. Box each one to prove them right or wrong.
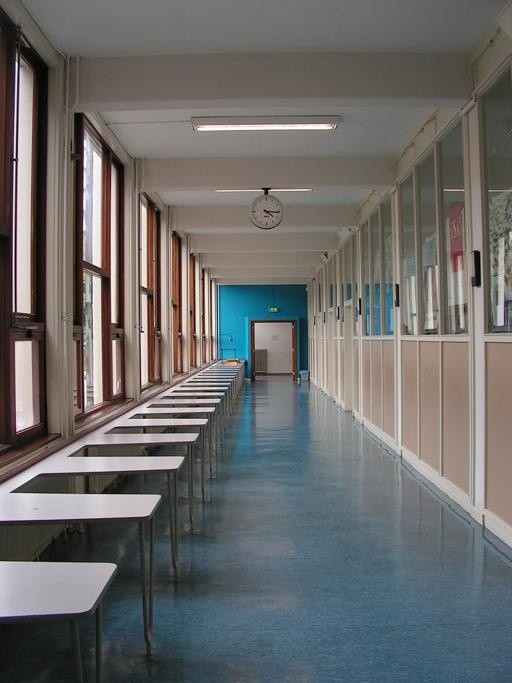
[0,555,118,680]
[2,491,163,660]
[52,427,209,542]
[2,449,185,596]
[95,359,247,485]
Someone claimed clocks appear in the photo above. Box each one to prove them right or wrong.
[248,188,283,230]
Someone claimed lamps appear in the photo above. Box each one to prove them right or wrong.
[190,115,341,132]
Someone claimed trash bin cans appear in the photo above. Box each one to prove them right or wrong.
[300,370,309,382]
